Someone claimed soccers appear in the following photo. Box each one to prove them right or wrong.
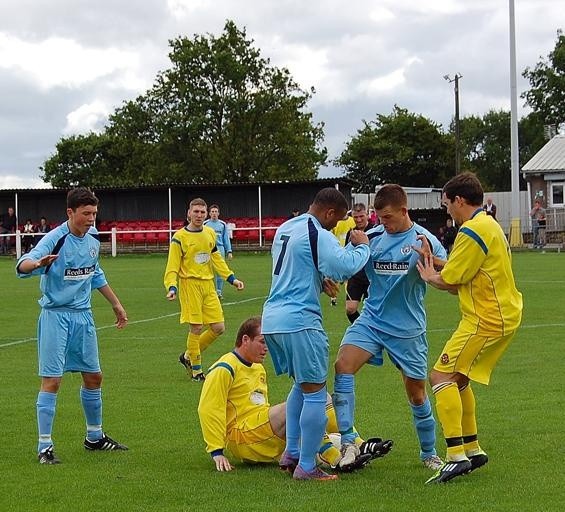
[315,433,342,469]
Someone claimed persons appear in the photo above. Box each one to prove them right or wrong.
[0,208,52,255]
[334,183,447,472]
[328,203,380,323]
[529,200,547,248]
[412,173,524,483]
[15,186,127,465]
[439,219,458,253]
[483,196,498,222]
[537,215,546,246]
[197,316,393,470]
[261,187,371,480]
[163,198,244,383]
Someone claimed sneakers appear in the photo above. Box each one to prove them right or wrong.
[419,451,489,483]
[39,445,57,464]
[180,352,193,377]
[278,438,394,480]
[190,373,205,382]
[331,299,337,306]
[218,292,224,302]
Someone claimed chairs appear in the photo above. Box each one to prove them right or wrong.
[0,215,291,254]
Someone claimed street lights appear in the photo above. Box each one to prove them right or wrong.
[442,73,465,176]
